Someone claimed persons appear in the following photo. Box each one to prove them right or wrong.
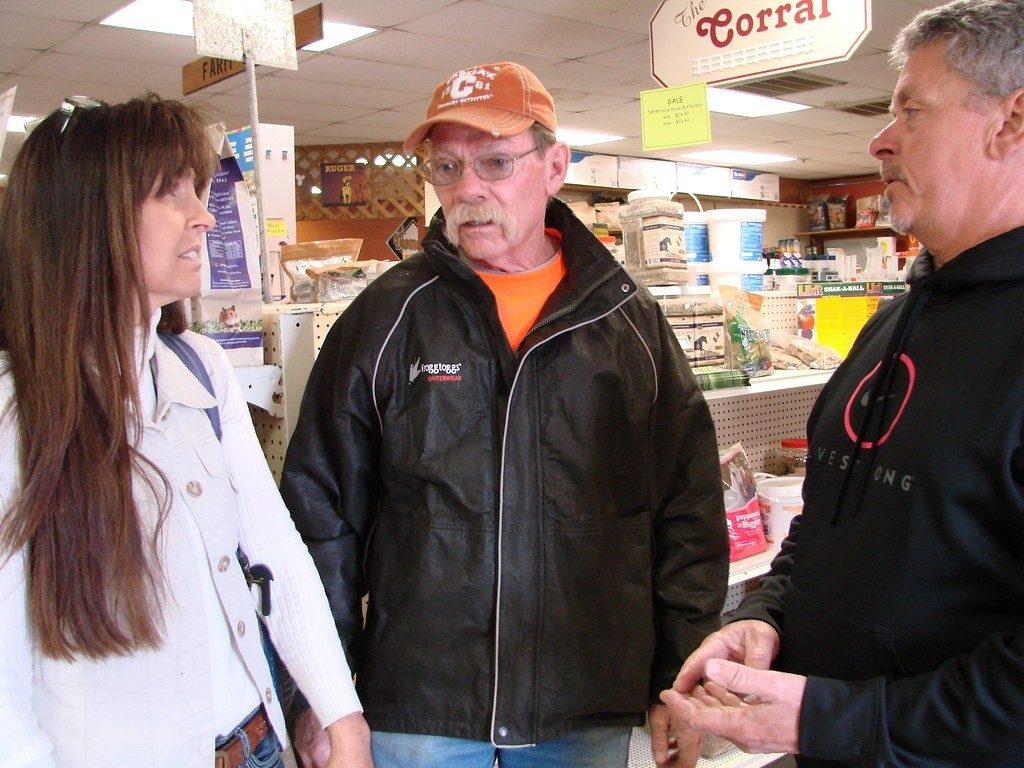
[0,93,376,768]
[259,61,732,768]
[661,0,1024,768]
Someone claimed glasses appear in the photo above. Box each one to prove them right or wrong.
[57,95,102,134]
[420,143,550,186]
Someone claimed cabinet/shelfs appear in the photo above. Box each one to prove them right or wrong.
[208,287,837,614]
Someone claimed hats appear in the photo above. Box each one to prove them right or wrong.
[403,62,557,153]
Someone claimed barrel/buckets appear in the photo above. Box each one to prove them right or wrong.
[678,210,707,286]
[705,208,768,291]
[618,189,690,286]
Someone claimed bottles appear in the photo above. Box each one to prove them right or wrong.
[776,438,808,476]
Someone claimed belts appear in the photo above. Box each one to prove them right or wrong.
[215,708,271,768]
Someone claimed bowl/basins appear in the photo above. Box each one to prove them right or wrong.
[281,239,406,303]
